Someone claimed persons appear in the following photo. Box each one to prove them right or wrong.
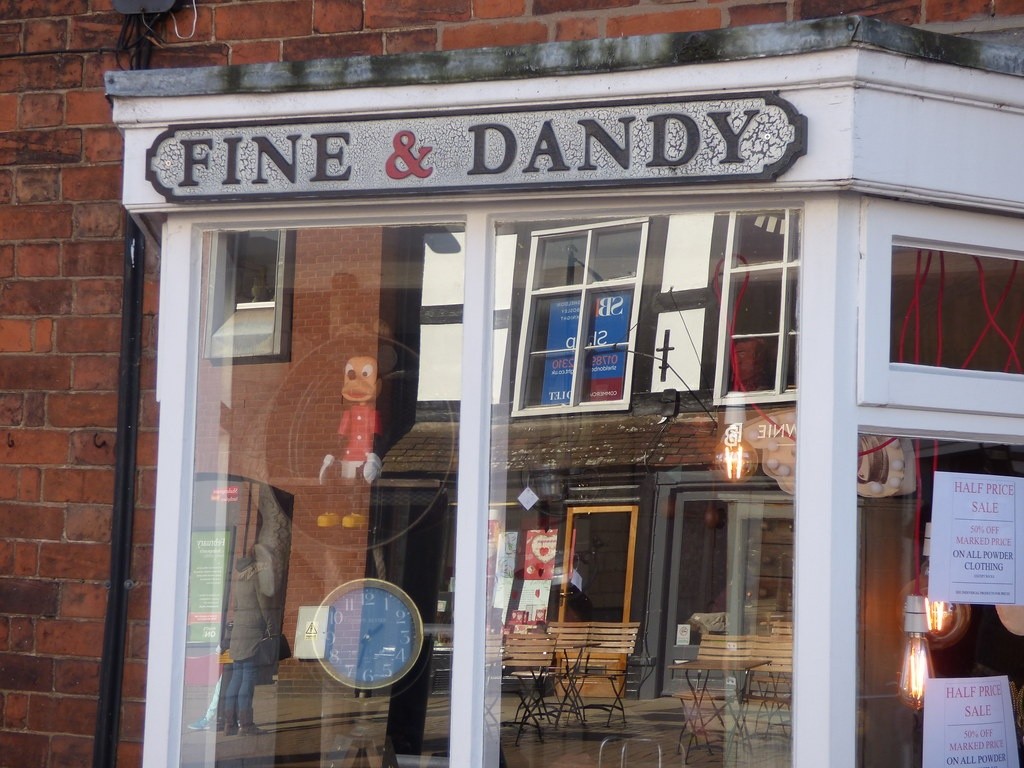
[189,542,292,734]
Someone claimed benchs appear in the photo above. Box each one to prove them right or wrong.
[505,623,793,763]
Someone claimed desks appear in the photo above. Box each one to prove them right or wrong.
[667,660,771,766]
[513,641,601,729]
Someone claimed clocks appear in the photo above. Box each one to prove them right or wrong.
[303,578,434,768]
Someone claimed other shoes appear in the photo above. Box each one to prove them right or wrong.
[187,717,212,730]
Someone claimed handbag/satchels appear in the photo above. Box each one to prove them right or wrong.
[254,630,291,666]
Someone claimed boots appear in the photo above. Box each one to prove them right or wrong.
[224,708,240,736]
[237,708,267,737]
